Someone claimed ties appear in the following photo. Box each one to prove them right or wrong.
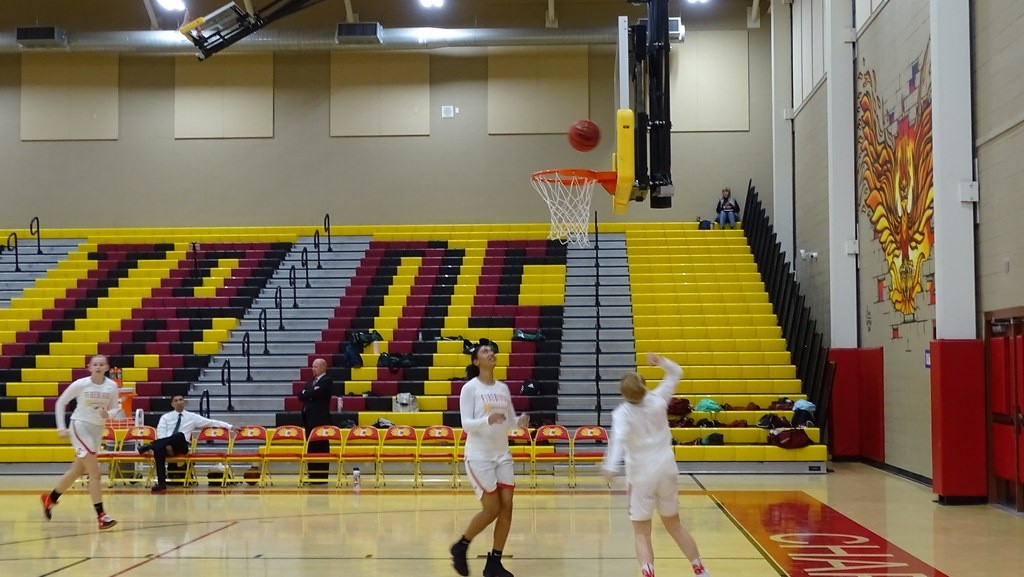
[172,414,182,435]
[313,378,318,386]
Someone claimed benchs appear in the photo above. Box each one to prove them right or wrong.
[0,221,828,463]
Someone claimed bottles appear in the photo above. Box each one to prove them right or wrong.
[338,394,344,412]
[352,467,360,492]
[374,337,379,355]
[419,331,422,341]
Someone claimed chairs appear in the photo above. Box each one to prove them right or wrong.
[72,426,609,489]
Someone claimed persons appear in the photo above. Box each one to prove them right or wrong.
[714,186,740,230]
[451,343,530,577]
[40,355,119,530]
[599,355,711,577]
[297,358,333,484]
[137,392,244,491]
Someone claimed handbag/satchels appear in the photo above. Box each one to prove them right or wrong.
[352,330,383,348]
[519,378,543,396]
[699,220,714,230]
[342,393,419,430]
[376,352,412,374]
[512,329,546,341]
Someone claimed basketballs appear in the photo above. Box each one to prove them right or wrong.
[569,120,600,151]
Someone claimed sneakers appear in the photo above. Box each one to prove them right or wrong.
[41,494,53,519]
[98,514,117,529]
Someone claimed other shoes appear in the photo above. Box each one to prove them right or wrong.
[642,566,655,577]
[451,543,469,577]
[693,563,709,577]
[152,483,167,492]
[483,552,514,577]
[138,445,147,455]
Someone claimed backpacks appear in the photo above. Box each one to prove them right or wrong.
[663,396,816,449]
